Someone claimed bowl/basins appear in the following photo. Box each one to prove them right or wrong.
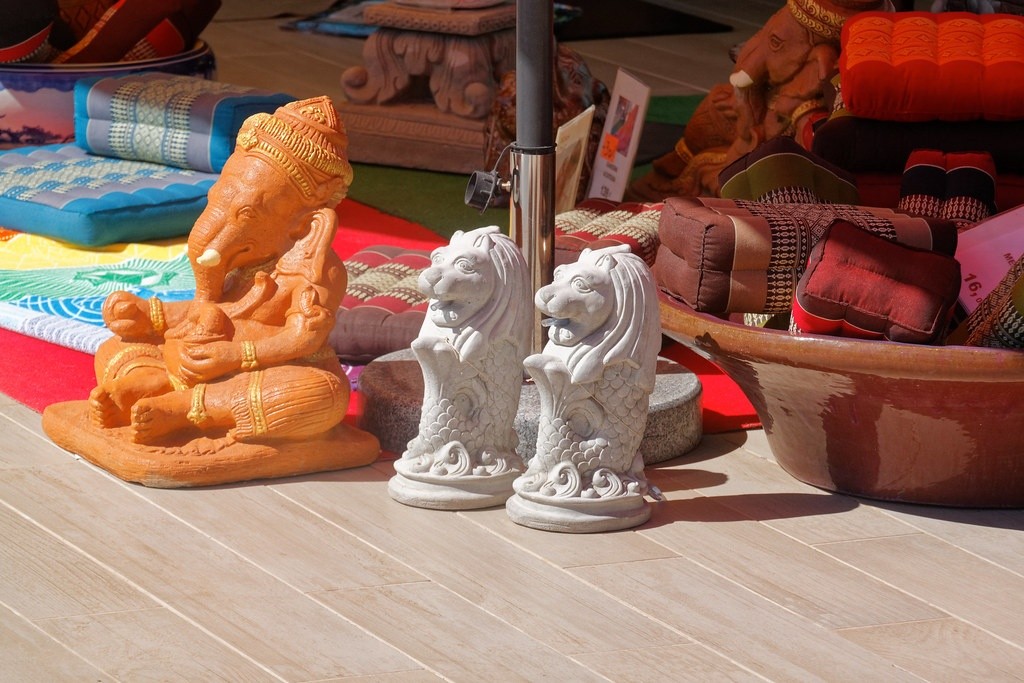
[659,292,1024,507]
[0,39,218,144]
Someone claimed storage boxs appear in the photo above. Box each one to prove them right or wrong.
[589,67,706,205]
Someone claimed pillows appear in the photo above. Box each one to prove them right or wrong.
[840,13,1024,122]
[73,68,296,173]
[554,137,1023,348]
[0,0,222,64]
